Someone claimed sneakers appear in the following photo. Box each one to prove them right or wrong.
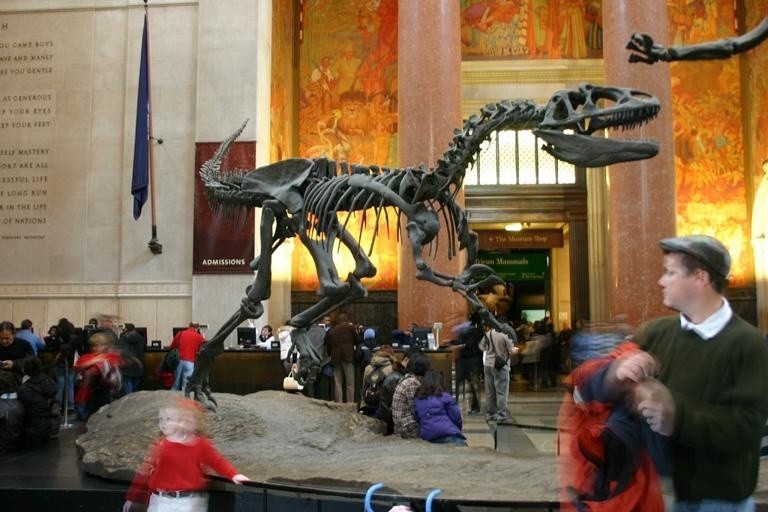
[468,408,507,422]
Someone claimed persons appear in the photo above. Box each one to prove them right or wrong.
[574,235,768,512]
[1,314,147,449]
[168,321,206,393]
[122,397,251,512]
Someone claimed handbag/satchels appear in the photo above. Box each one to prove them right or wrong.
[283,369,305,391]
[494,356,506,371]
[160,348,181,374]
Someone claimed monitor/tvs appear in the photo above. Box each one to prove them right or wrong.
[237,327,256,348]
[135,327,147,344]
[173,327,188,338]
[413,327,432,346]
[15,328,33,334]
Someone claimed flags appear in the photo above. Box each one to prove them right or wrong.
[129,14,149,221]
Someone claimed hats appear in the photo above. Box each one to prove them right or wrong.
[658,234,731,275]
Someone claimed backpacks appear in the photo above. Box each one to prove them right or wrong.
[363,367,386,404]
